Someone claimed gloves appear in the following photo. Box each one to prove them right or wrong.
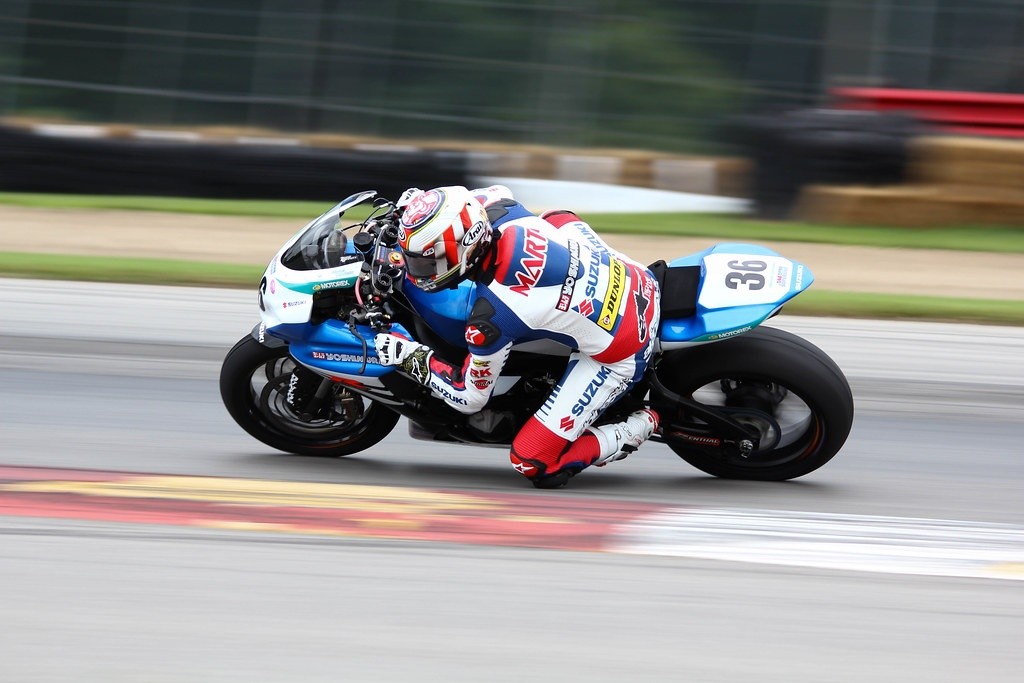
[374,333,423,366]
[396,188,425,207]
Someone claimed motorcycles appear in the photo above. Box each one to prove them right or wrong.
[220,191,855,481]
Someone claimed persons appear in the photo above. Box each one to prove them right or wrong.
[373,182,660,491]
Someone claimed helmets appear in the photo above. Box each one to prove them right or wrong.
[398,186,494,292]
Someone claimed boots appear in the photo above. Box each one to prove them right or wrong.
[597,409,660,467]
